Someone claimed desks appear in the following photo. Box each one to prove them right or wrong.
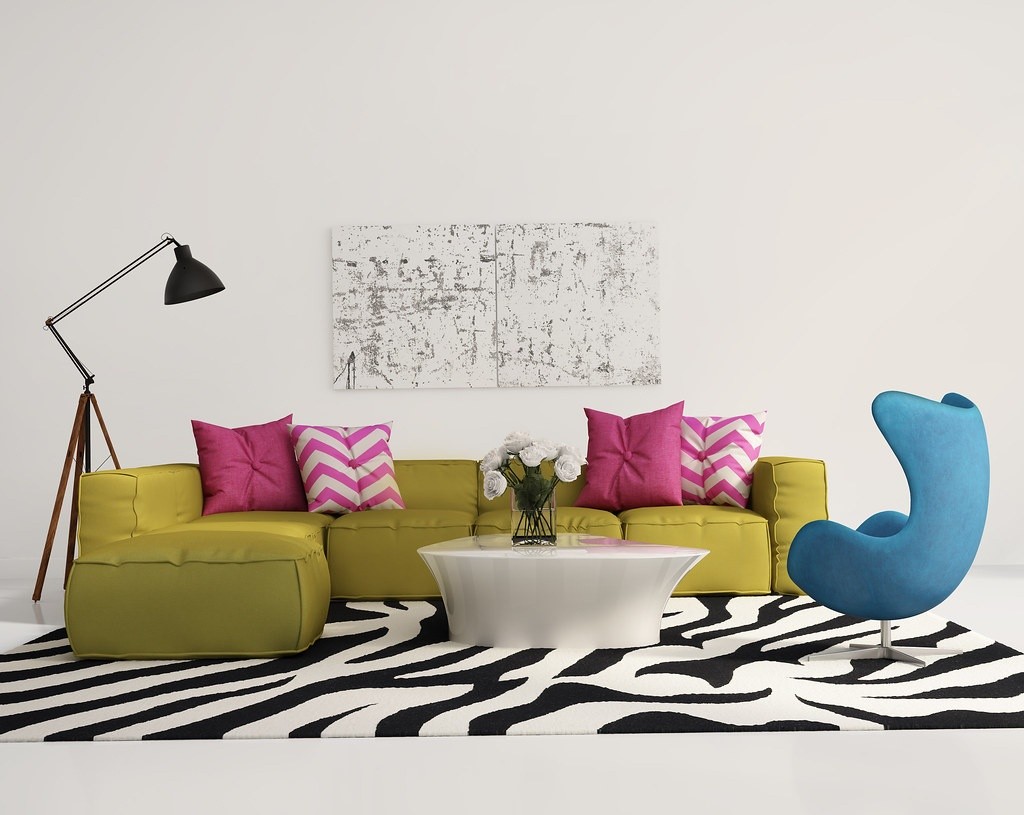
[417,531,711,650]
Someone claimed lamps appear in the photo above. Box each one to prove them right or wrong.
[30,233,225,604]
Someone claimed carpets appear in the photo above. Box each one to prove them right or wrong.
[0,596,1024,744]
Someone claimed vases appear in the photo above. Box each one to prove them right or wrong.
[512,488,556,547]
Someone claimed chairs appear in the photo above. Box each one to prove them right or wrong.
[786,391,991,669]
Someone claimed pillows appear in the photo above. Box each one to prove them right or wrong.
[287,419,405,515]
[572,399,686,507]
[681,410,769,510]
[190,413,306,515]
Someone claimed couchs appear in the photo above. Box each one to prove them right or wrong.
[66,457,828,656]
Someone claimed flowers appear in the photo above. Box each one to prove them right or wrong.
[479,431,588,542]
